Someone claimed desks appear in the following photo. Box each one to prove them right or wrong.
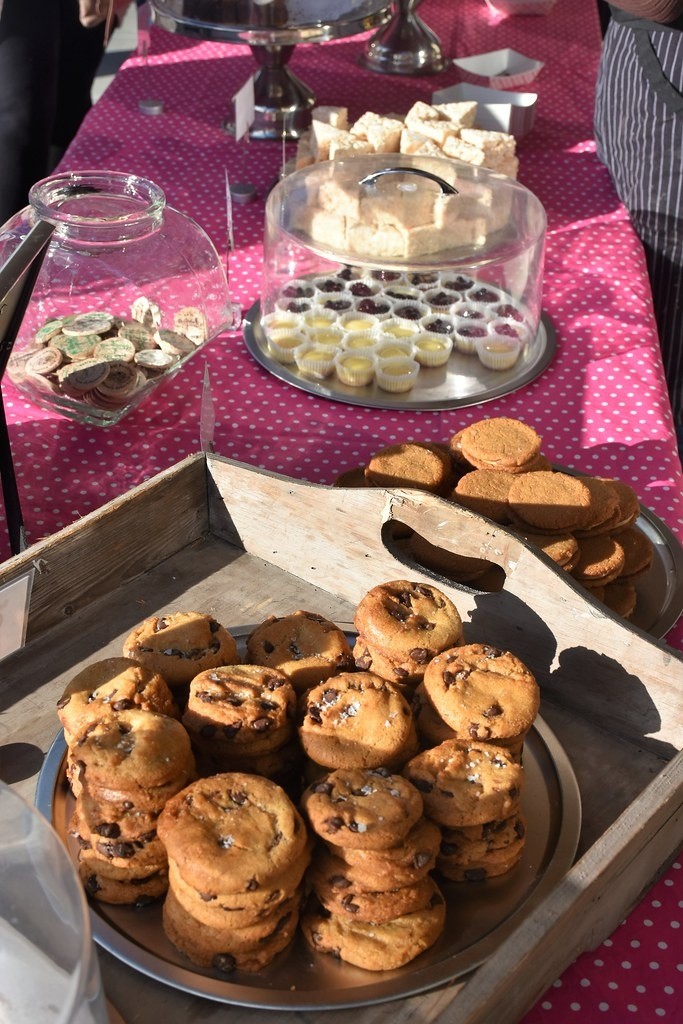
[1,0,683,1024]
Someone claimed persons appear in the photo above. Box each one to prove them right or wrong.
[0,0,130,230]
[593,0,683,474]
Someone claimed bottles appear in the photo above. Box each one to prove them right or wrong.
[0,169,233,429]
[1,784,109,1024]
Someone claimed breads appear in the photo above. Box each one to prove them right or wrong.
[286,99,518,259]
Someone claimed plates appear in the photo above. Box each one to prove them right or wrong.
[30,622,582,1012]
[329,458,683,642]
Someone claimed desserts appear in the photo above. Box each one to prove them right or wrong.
[259,264,531,393]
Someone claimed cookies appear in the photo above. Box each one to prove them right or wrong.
[363,415,653,622]
[58,579,540,975]
